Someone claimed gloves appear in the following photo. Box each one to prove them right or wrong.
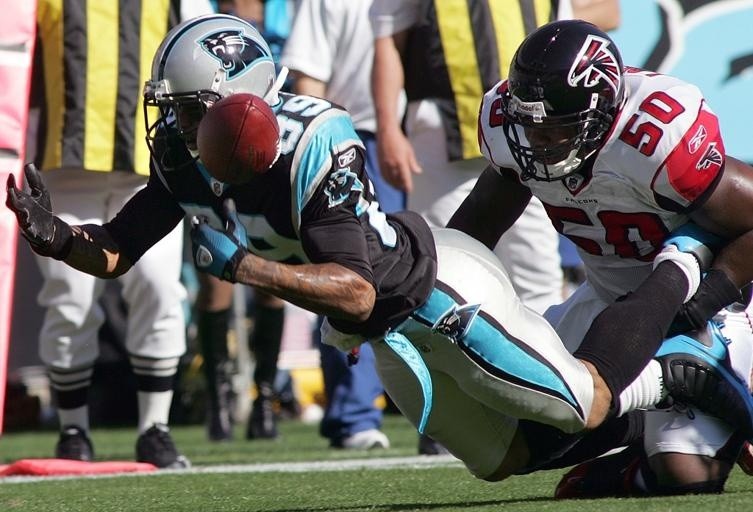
[191,198,248,283]
[6,162,53,248]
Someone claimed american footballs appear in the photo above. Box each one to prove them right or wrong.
[197,93,280,185]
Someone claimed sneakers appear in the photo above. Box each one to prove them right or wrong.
[210,399,275,439]
[655,225,753,476]
[58,426,93,462]
[344,429,389,448]
[555,445,640,498]
[136,423,188,467]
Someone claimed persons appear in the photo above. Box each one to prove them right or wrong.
[448,20,753,500]
[35,0,216,471]
[366,0,620,456]
[5,11,753,483]
[198,0,298,439]
[278,0,405,451]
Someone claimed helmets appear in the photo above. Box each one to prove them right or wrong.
[501,20,625,181]
[144,14,277,165]
